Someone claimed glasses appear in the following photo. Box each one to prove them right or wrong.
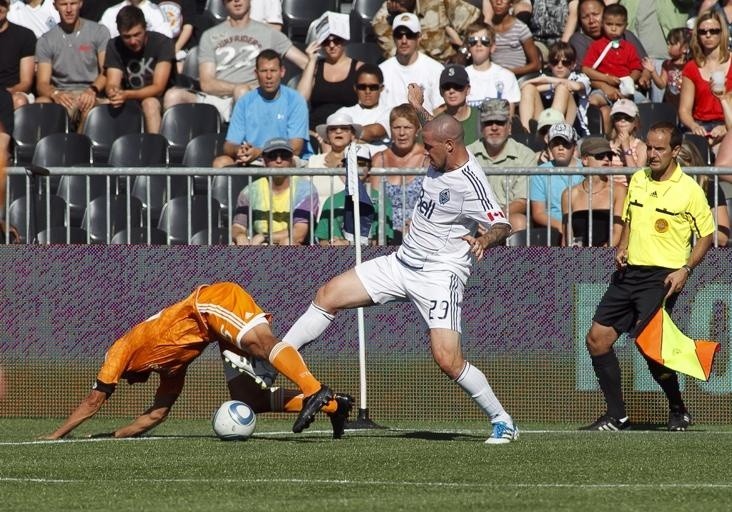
[322,38,343,46]
[697,29,720,36]
[550,58,572,68]
[395,32,417,39]
[467,37,490,47]
[356,84,379,92]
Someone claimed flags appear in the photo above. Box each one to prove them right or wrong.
[632,308,722,382]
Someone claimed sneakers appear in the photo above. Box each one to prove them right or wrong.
[668,409,693,432]
[579,414,634,432]
[223,350,279,391]
[331,392,353,439]
[484,416,520,444]
[292,386,333,434]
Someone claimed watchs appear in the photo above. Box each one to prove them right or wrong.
[681,264,693,278]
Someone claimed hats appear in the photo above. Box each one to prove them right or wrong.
[316,114,362,137]
[261,139,293,155]
[341,143,370,162]
[581,136,616,156]
[480,98,510,123]
[440,65,471,89]
[537,107,581,145]
[392,13,420,35]
[608,98,640,119]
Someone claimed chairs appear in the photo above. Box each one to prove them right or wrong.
[1,1,717,246]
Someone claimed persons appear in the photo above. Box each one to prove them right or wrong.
[1,0,731,245]
[40,281,356,440]
[221,113,520,446]
[576,120,717,431]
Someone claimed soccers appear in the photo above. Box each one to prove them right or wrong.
[212,401,257,441]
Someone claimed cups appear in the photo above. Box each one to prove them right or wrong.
[709,71,725,94]
